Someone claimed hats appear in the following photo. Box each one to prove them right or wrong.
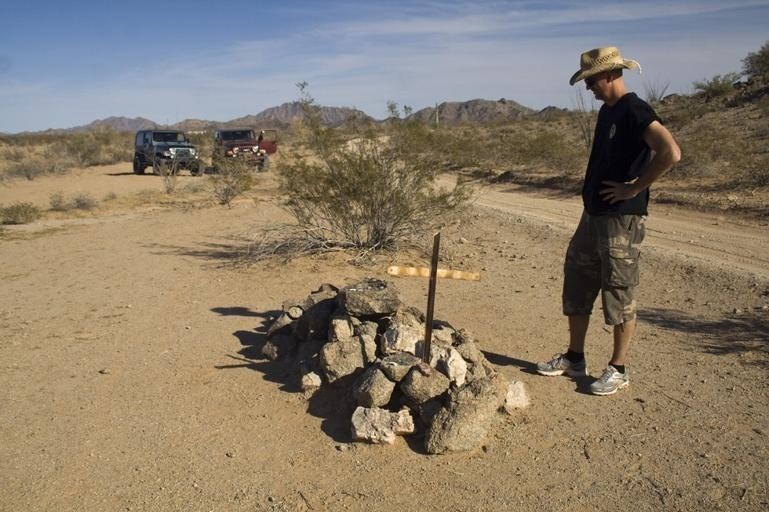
[569,46,640,86]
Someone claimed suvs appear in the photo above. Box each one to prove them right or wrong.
[213,127,278,172]
[134,128,199,177]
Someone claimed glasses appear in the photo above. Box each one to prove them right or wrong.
[585,76,608,88]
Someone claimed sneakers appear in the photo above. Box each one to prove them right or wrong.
[536,351,587,378]
[589,366,630,397]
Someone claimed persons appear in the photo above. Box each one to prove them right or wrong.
[534,46,682,396]
